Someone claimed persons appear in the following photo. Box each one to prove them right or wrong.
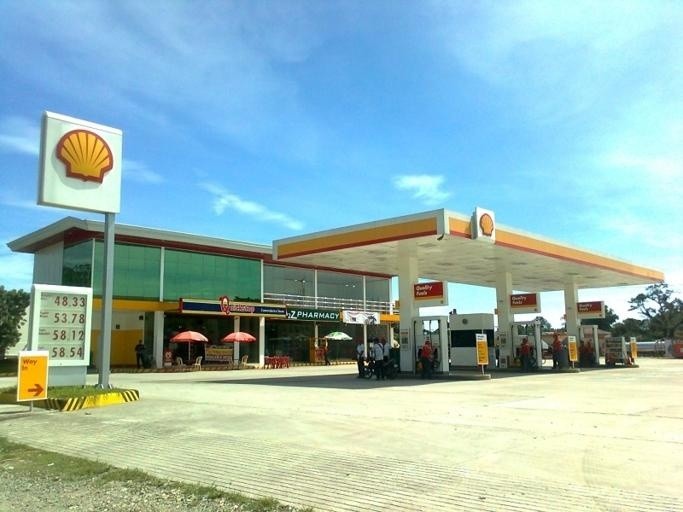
[577,340,596,368]
[519,338,531,371]
[355,336,393,381]
[322,338,331,366]
[550,332,563,370]
[135,339,147,370]
[421,340,434,380]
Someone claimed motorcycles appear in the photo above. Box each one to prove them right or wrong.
[361,357,399,380]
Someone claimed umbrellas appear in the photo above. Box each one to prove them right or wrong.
[223,331,256,360]
[170,330,208,360]
[323,331,353,365]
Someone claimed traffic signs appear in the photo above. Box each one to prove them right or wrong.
[16,351,49,402]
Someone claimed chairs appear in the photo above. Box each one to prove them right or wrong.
[228,355,249,367]
[176,355,203,372]
